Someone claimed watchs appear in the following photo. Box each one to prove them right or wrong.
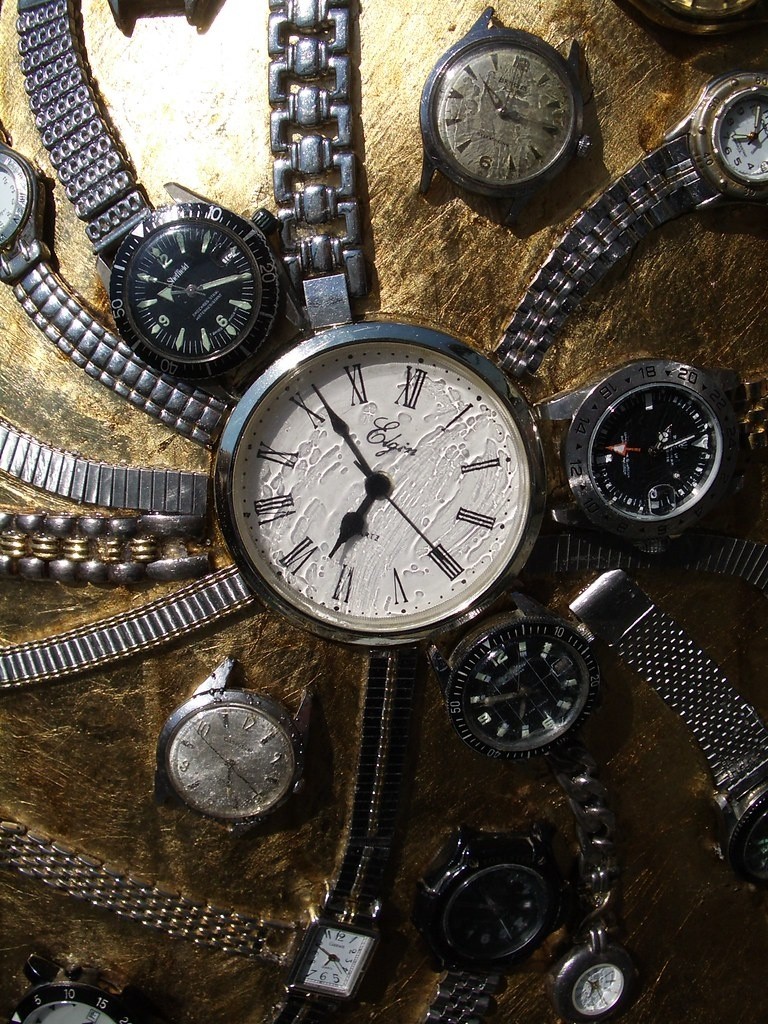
[429,592,629,1024]
[12,0,306,404]
[570,571,768,888]
[213,0,549,648]
[417,5,592,218]
[537,359,768,556]
[0,953,160,1024]
[493,65,768,374]
[257,650,419,1024]
[608,0,767,57]
[152,658,318,840]
[409,822,568,1024]
